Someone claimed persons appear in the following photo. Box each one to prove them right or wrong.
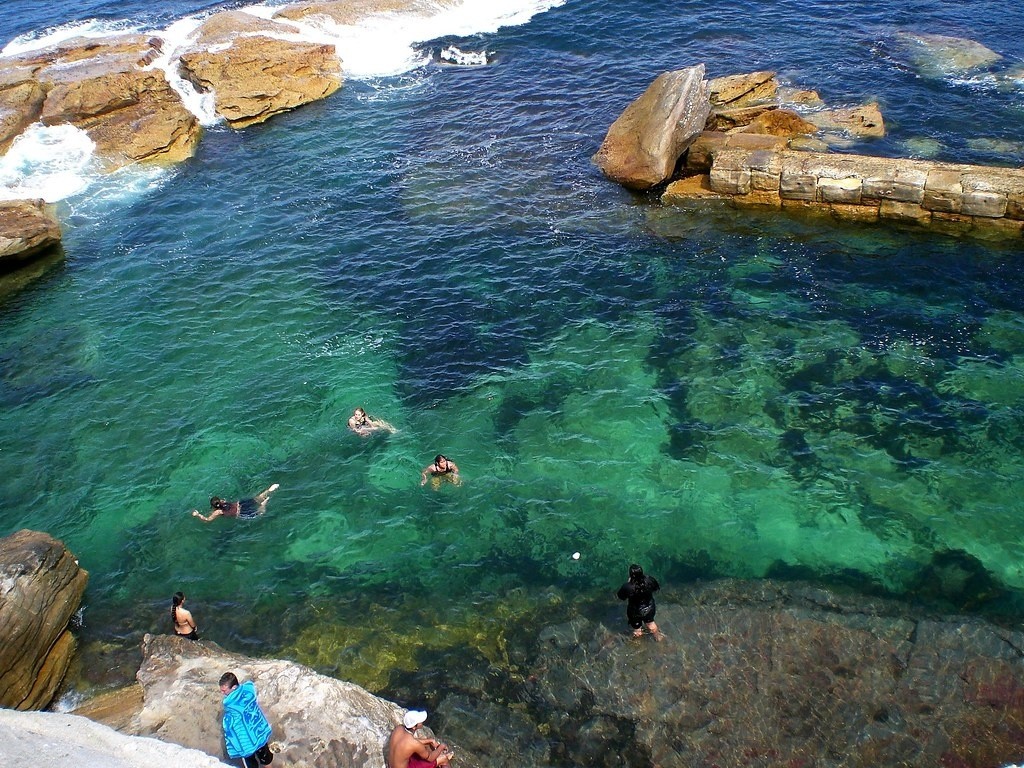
[192,483,279,522]
[219,672,273,768]
[170,591,198,640]
[617,564,660,630]
[431,455,454,471]
[390,710,454,768]
[347,407,374,428]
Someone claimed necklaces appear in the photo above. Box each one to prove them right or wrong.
[404,728,413,735]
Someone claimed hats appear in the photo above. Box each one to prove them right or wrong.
[403,710,427,728]
